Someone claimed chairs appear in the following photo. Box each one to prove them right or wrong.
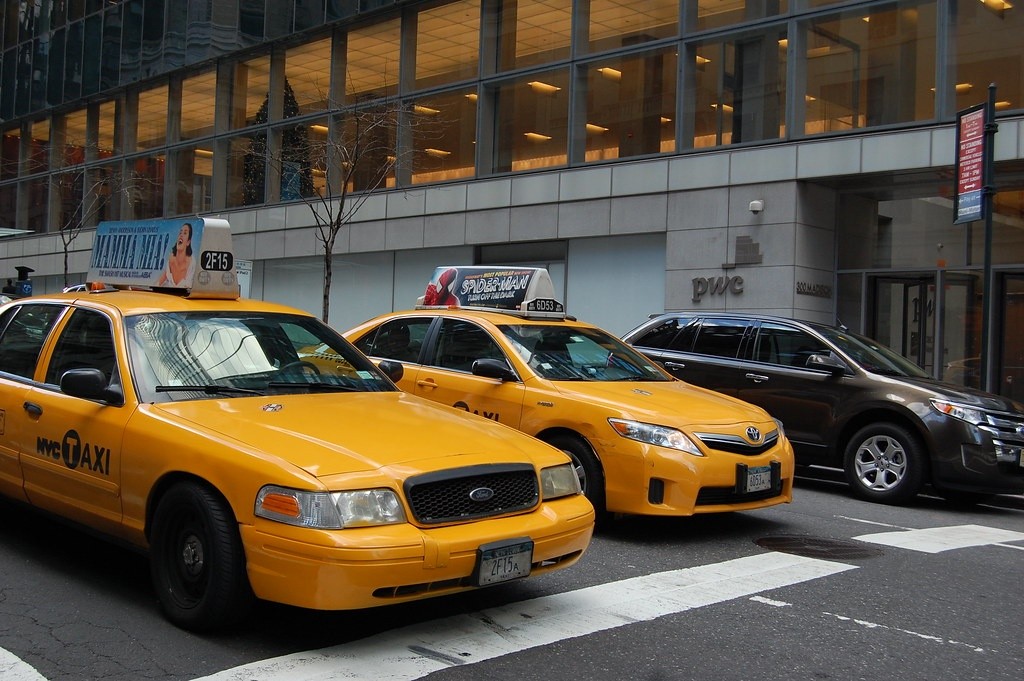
[448,329,487,372]
[757,337,771,361]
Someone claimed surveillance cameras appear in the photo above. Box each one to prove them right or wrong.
[748,200,762,215]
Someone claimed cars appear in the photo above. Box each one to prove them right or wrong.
[607,312,1024,505]
[62,285,113,292]
[296,307,795,536]
[0,289,595,633]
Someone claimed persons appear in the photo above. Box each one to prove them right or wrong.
[383,326,418,363]
[2,278,15,294]
[159,223,196,288]
